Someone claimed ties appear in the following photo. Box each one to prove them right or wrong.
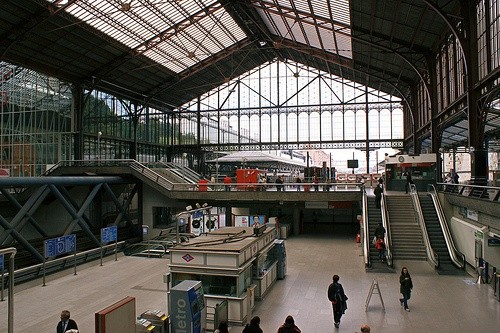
[62,321,66,333]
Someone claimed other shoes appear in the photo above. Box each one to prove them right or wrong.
[406,309,410,312]
[333,322,340,328]
[400,298,402,306]
[379,260,383,262]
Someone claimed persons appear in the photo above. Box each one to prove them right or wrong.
[444,169,459,193]
[198,175,208,191]
[361,325,370,333]
[214,322,229,333]
[405,172,416,194]
[56,311,79,333]
[211,175,215,190]
[374,223,386,263]
[224,175,232,191]
[357,175,367,194]
[328,275,344,328]
[260,172,337,192]
[277,315,301,333]
[399,267,413,312]
[311,211,317,229]
[374,185,383,208]
[242,316,263,333]
[251,216,260,227]
[378,177,384,184]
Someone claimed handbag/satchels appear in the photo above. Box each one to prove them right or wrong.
[379,239,385,249]
[372,236,377,245]
